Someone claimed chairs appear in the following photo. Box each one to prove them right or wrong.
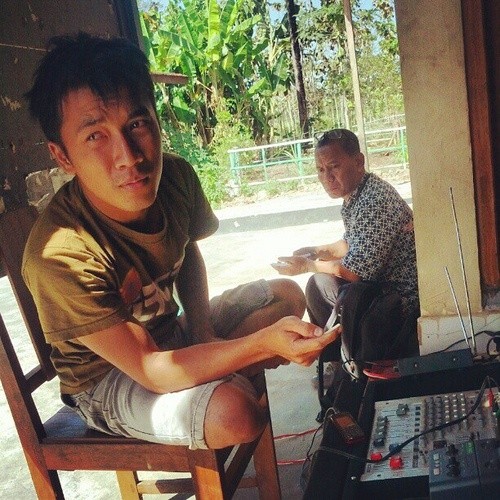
[0,205,280,500]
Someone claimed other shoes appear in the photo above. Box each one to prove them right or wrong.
[311,360,340,389]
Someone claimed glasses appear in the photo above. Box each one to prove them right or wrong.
[313,130,358,143]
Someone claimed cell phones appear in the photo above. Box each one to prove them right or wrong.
[330,410,366,445]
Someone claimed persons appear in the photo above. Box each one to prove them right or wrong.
[270,129,421,388]
[20,30,342,450]
